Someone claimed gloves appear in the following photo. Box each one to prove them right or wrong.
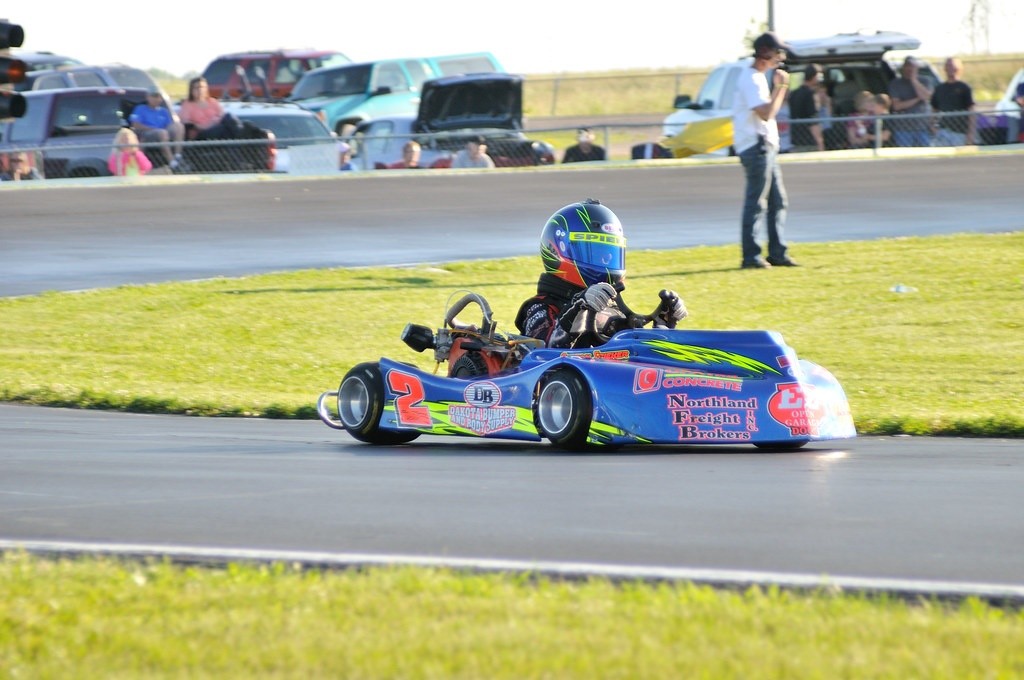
[573,282,617,311]
[653,290,689,325]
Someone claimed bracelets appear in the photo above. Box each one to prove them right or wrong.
[779,84,788,87]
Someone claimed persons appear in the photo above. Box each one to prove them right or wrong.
[1012,81,1024,142]
[403,141,421,168]
[788,62,823,152]
[108,127,152,176]
[886,55,931,147]
[846,91,896,148]
[451,135,493,168]
[3,151,42,181]
[734,32,800,268]
[339,142,356,171]
[928,58,977,146]
[128,77,252,170]
[562,129,605,163]
[515,197,687,350]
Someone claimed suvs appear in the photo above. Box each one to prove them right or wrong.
[0,51,556,179]
[664,30,1024,159]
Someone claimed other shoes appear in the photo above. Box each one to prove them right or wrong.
[771,260,801,267]
[741,258,771,268]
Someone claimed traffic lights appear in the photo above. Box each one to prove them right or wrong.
[0,20,29,120]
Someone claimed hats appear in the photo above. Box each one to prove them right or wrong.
[904,57,917,65]
[753,32,792,52]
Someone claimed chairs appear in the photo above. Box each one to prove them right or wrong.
[834,78,859,114]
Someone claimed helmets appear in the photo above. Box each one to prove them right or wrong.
[539,203,627,293]
